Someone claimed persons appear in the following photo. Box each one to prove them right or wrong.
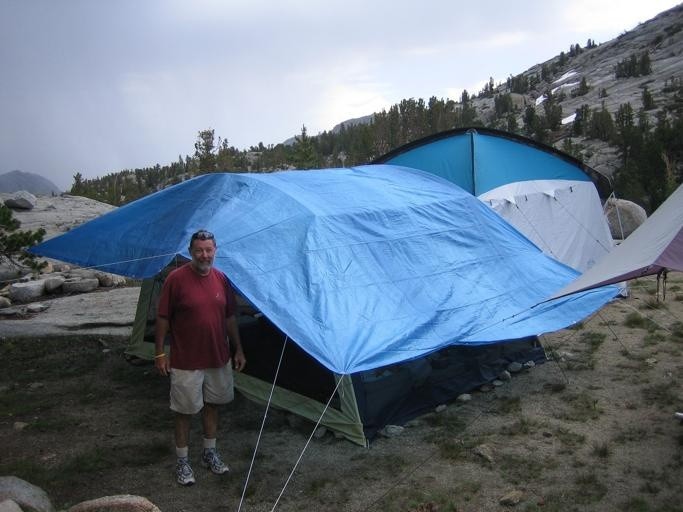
[152,230,248,486]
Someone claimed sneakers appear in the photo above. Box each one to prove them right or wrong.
[175,456,196,486]
[200,447,230,475]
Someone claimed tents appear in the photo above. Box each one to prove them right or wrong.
[371,126,629,300]
[25,163,622,447]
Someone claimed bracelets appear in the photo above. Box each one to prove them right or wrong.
[153,353,166,359]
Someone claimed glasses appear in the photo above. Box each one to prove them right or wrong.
[196,232,212,240]
